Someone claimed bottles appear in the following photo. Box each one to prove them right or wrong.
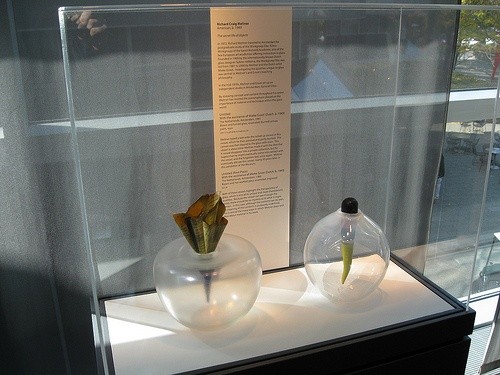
[153,232,264,331]
[303,197,390,305]
